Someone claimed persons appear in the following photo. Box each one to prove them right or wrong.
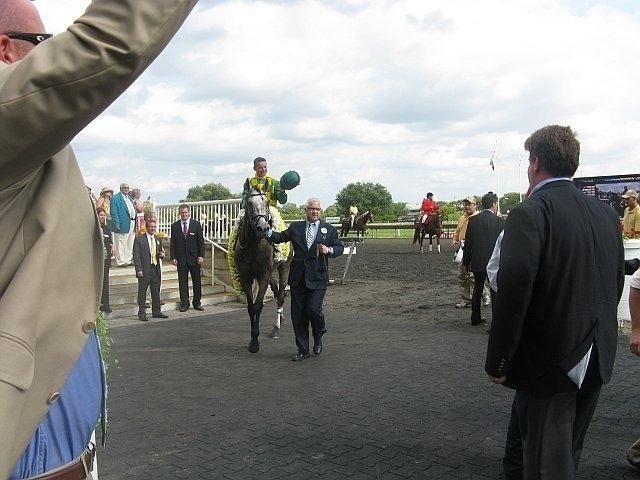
[87,183,232,321]
[0,0,198,480]
[452,196,491,309]
[350,202,359,229]
[486,230,524,480]
[462,192,505,325]
[266,198,344,362]
[620,190,640,238]
[421,193,439,229]
[627,265,640,470]
[241,157,289,263]
[484,124,626,480]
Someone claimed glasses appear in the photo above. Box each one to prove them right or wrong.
[4,29,58,48]
[307,207,322,212]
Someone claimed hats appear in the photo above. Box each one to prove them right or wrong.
[426,192,433,197]
[99,185,115,194]
[462,195,477,204]
[280,169,300,191]
[620,189,638,200]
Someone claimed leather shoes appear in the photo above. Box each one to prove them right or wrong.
[293,350,310,362]
[312,337,323,355]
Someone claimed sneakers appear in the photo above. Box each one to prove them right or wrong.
[484,295,492,307]
[152,311,170,319]
[471,317,486,327]
[453,298,472,309]
[193,305,204,312]
[180,304,190,312]
[137,311,148,323]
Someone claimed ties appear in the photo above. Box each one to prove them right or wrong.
[183,221,188,240]
[307,223,315,251]
[150,237,157,266]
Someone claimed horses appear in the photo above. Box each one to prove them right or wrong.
[412,210,444,256]
[339,210,373,243]
[233,177,293,353]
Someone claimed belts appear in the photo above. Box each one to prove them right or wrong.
[25,442,99,480]
